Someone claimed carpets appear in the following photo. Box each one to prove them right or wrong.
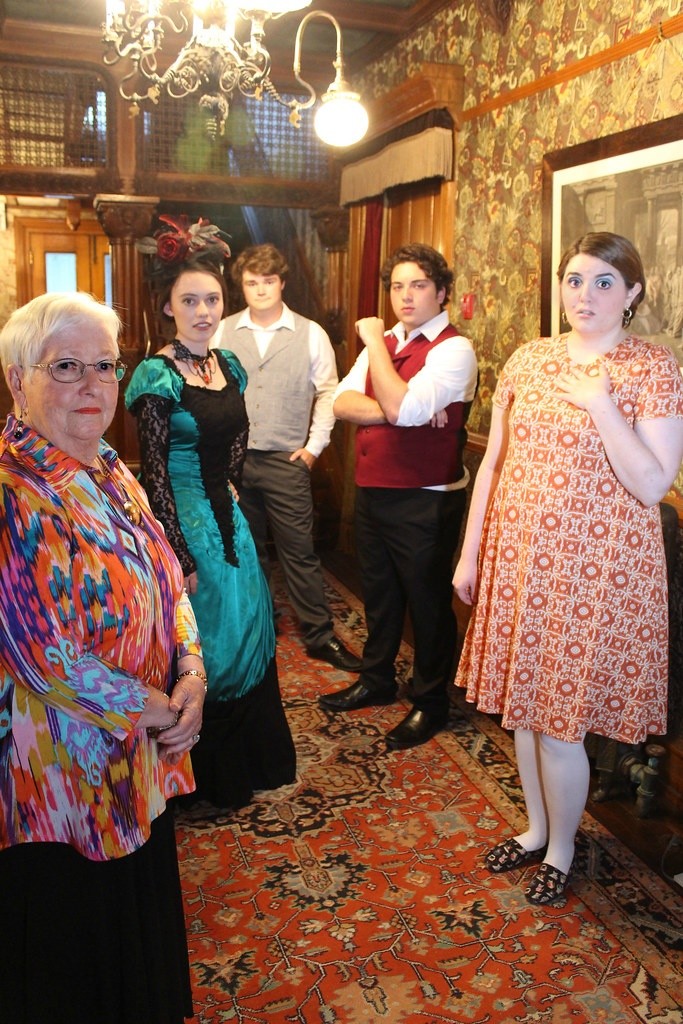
[165,538,683,1024]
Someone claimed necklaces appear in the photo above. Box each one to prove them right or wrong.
[169,338,216,384]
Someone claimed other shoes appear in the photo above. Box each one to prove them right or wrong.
[485,833,548,872]
[526,861,573,905]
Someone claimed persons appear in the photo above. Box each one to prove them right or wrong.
[0,291,206,1024]
[123,216,296,805]
[208,245,362,672]
[452,232,683,903]
[318,242,480,747]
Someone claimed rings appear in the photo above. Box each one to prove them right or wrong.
[191,735,200,744]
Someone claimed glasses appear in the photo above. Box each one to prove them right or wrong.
[19,357,127,383]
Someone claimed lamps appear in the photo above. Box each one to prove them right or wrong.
[99,1,369,145]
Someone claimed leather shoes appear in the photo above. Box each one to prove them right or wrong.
[385,704,450,748]
[319,681,398,709]
[309,634,365,671]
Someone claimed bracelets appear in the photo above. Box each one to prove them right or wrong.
[176,670,208,692]
[148,694,181,736]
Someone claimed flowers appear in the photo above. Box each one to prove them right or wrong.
[139,210,234,264]
